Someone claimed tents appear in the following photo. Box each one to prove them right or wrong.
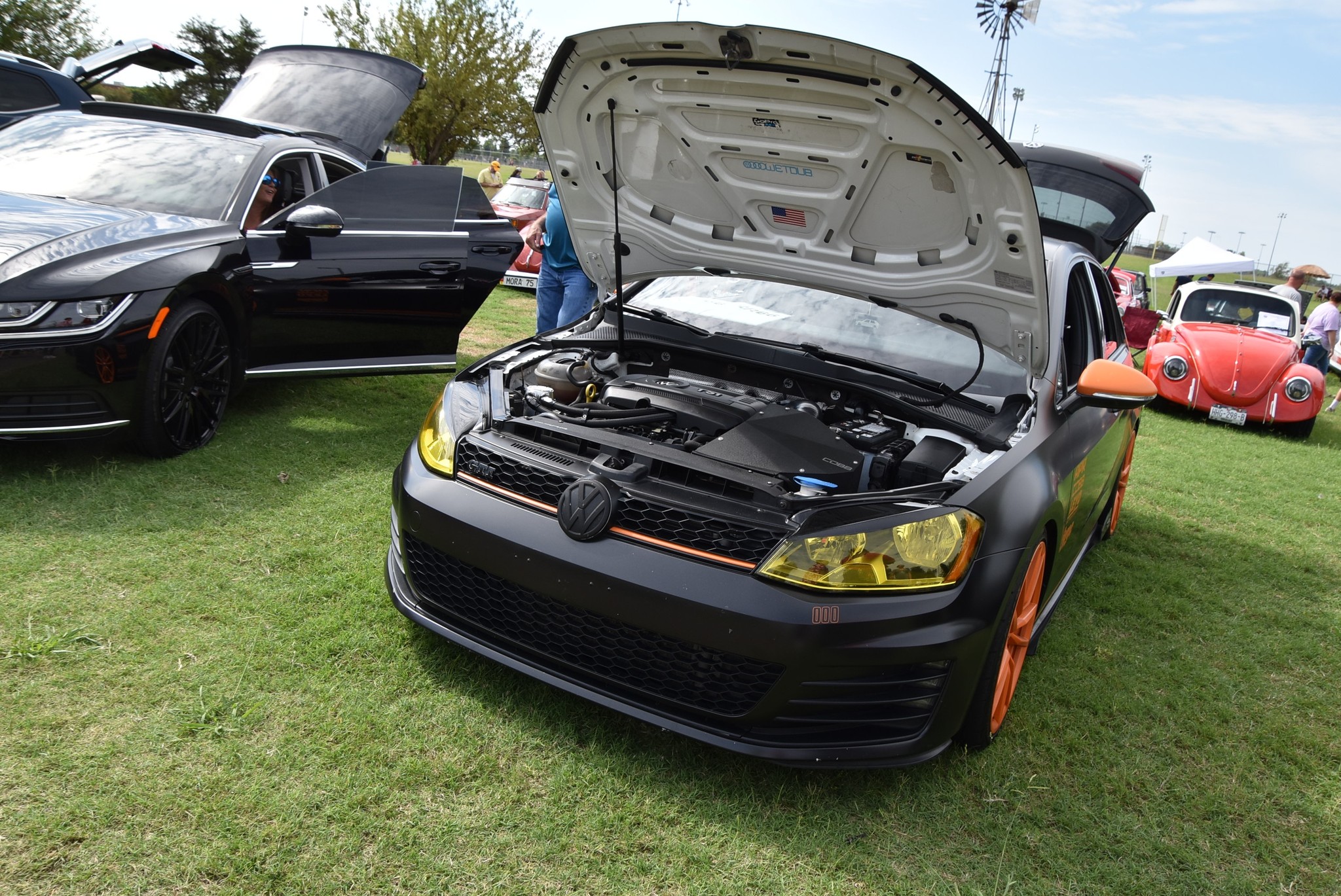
[1149,236,1255,312]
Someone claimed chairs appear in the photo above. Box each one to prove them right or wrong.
[1315,287,1333,301]
[266,171,292,230]
[1122,305,1162,368]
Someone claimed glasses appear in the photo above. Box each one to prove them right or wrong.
[262,175,282,188]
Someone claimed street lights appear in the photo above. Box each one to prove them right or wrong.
[1126,154,1152,254]
[1031,126,1039,141]
[1181,232,1187,248]
[1257,244,1266,270]
[1265,212,1287,277]
[1236,232,1246,254]
[1207,231,1216,242]
[1007,87,1024,139]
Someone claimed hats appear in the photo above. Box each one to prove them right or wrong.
[491,161,501,171]
[538,170,545,179]
[516,167,522,172]
[1289,269,1306,281]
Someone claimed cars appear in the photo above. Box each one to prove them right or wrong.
[0,39,524,462]
[1141,281,1325,440]
[383,23,1160,776]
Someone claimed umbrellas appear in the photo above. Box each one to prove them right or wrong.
[1293,265,1330,291]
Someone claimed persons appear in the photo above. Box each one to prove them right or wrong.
[1300,292,1341,397]
[510,167,522,178]
[470,154,514,165]
[1325,388,1341,412]
[241,169,281,237]
[1099,268,1121,299]
[526,181,598,333]
[412,159,419,165]
[478,161,503,201]
[1198,274,1215,281]
[533,169,545,179]
[1269,268,1305,314]
[1170,275,1194,296]
[1313,286,1329,303]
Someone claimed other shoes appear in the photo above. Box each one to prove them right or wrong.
[1324,407,1336,413]
[1325,394,1336,398]
